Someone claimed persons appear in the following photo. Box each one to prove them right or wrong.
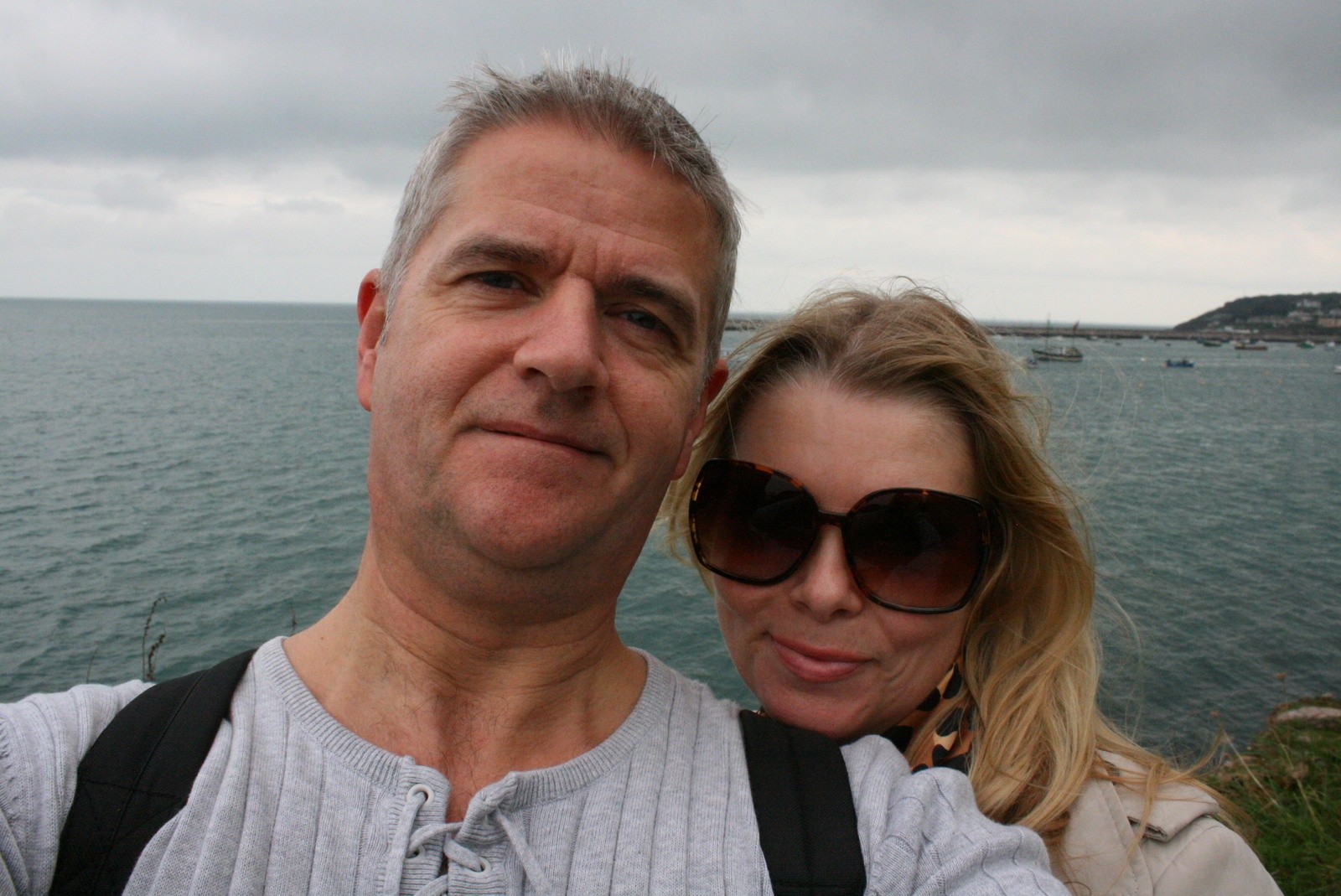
[0,40,1069,895]
[656,275,1284,896]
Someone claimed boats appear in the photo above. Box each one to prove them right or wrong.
[1031,311,1082,361]
[1165,356,1194,368]
[1197,330,1316,352]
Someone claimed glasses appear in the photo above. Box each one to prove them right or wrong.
[687,457,996,613]
